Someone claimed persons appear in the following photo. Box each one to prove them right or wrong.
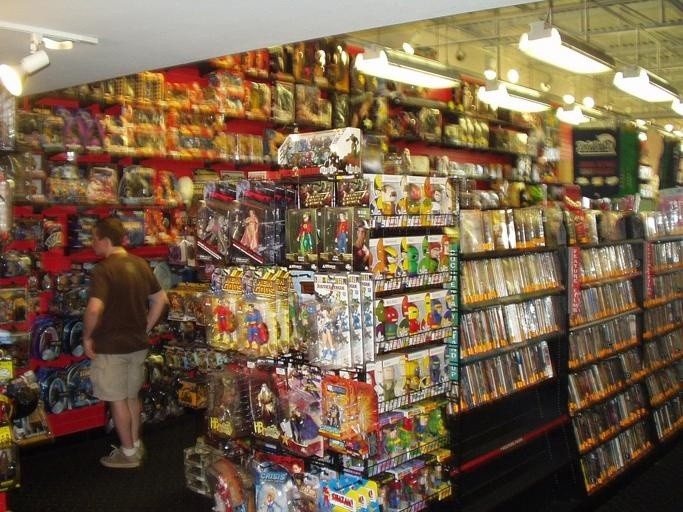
[333,211,350,256]
[324,401,342,432]
[242,303,269,357]
[198,209,227,256]
[208,298,236,345]
[296,301,316,347]
[294,211,316,258]
[236,208,261,254]
[254,381,284,441]
[315,305,342,364]
[283,390,321,442]
[82,217,168,469]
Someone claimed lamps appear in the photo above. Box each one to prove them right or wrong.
[353,32,461,92]
[513,1,617,80]
[0,35,74,98]
[474,17,551,116]
[612,27,679,102]
[517,80,614,80]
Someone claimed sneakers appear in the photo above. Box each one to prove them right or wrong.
[99,440,145,468]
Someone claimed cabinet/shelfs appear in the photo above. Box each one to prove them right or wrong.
[446,207,580,511]
[566,210,659,497]
[1,41,573,446]
[205,215,462,512]
[639,209,682,457]
